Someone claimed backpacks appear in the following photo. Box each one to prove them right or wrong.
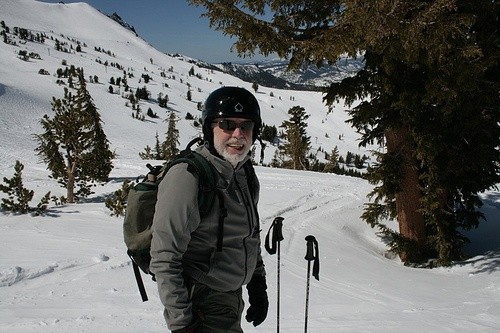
[123,137,259,301]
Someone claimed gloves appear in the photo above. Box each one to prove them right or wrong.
[245,287,268,326]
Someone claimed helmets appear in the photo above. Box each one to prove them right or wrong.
[201,85,262,149]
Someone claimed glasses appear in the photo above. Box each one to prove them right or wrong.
[212,116,255,134]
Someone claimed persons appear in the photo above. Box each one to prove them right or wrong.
[148,87,269,333]
